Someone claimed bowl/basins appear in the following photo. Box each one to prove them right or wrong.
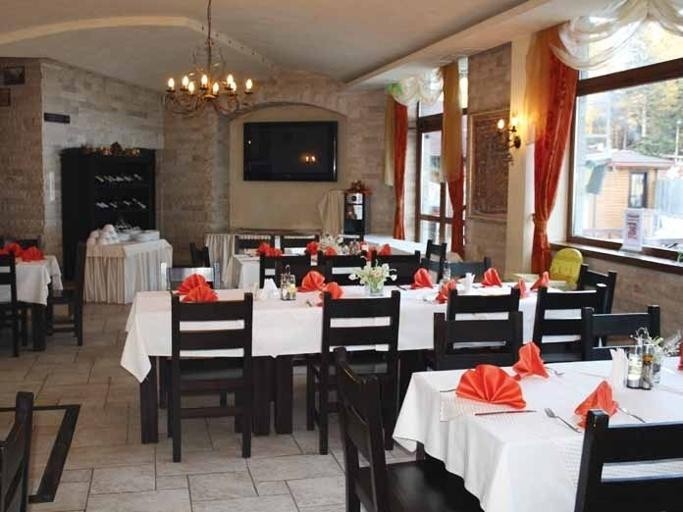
[87,224,119,245]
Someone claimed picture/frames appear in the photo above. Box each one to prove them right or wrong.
[466,107,510,225]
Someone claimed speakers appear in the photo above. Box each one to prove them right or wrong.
[344,192,372,245]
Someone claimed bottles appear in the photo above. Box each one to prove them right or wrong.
[444,261,451,280]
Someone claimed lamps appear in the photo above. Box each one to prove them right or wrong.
[161,2,255,117]
[490,116,523,153]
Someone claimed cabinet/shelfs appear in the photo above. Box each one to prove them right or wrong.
[61,146,156,290]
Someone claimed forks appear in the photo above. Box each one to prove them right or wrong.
[544,408,581,435]
[543,366,565,376]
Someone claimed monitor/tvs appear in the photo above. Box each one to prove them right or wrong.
[242,120,339,183]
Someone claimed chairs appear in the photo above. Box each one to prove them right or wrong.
[259,252,311,289]
[417,286,521,372]
[333,347,484,512]
[280,236,320,254]
[0,251,29,357]
[0,391,34,512]
[572,408,683,512]
[327,233,365,249]
[423,240,448,286]
[0,234,43,252]
[167,290,254,464]
[434,310,524,372]
[532,283,607,362]
[234,236,275,254]
[189,242,217,289]
[306,289,401,455]
[44,243,88,347]
[371,250,420,286]
[577,263,618,315]
[581,304,661,360]
[317,250,368,286]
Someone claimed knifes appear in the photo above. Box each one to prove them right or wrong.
[474,409,538,416]
